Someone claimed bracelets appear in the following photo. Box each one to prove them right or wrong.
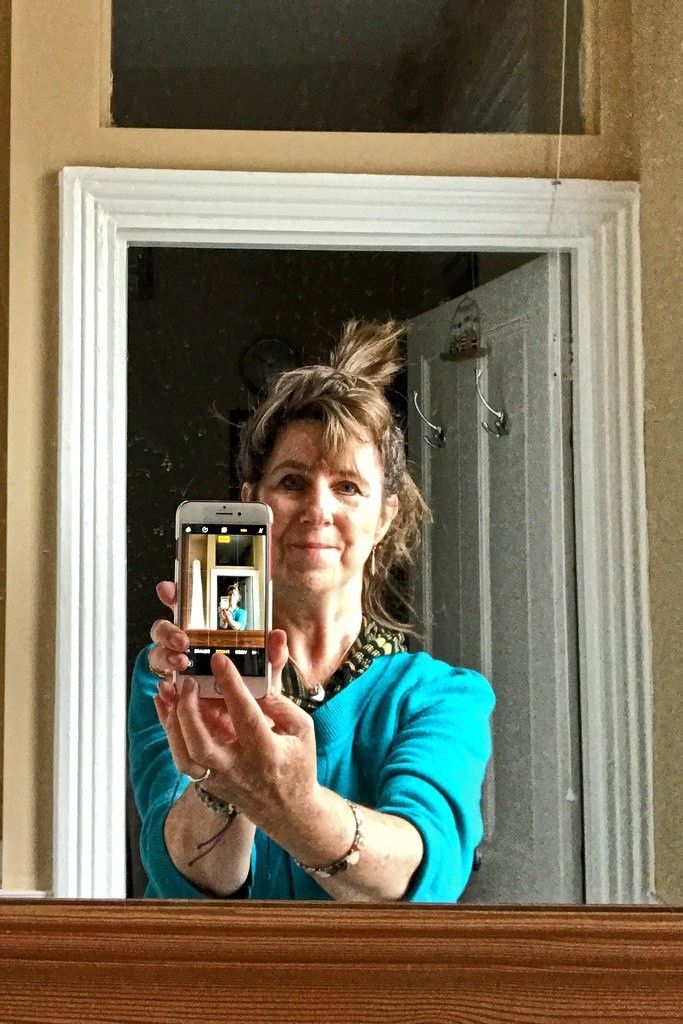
[292,799,367,877]
[184,781,244,866]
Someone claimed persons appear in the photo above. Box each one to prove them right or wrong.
[220,583,247,631]
[125,317,497,902]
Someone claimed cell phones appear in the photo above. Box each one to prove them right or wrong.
[173,499,274,700]
[220,596,230,611]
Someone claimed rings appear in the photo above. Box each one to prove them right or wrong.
[188,769,210,782]
[148,648,165,678]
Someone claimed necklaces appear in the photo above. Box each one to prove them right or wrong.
[288,637,356,702]
